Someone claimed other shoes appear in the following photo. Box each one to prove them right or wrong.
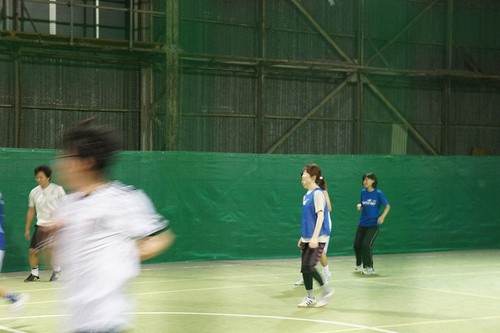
[24,274,40,282]
[353,264,375,276]
[49,270,62,282]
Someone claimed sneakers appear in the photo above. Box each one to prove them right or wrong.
[317,288,335,307]
[297,296,317,307]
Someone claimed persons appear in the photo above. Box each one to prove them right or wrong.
[298,164,333,307]
[0,193,22,303]
[295,178,331,285]
[52,127,175,332]
[24,165,66,281]
[355,173,389,275]
